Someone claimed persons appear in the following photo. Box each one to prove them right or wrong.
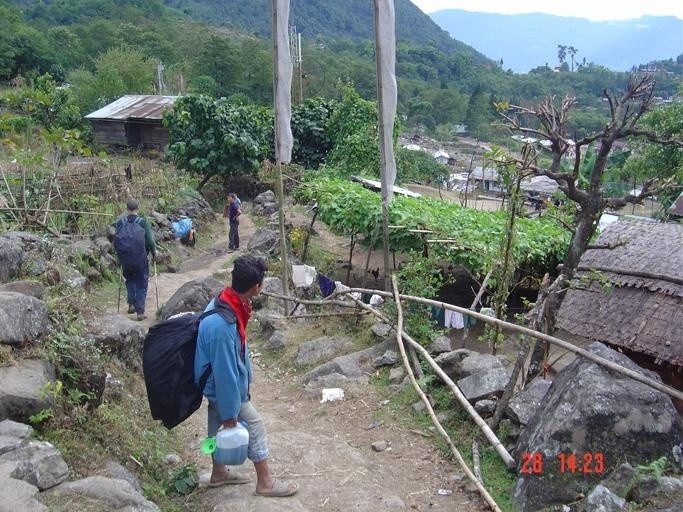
[193,255,299,497]
[180,226,197,249]
[227,192,242,253]
[232,192,242,210]
[112,198,158,321]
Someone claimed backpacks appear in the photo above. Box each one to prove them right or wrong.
[141,309,236,430]
[113,216,147,272]
[184,227,196,247]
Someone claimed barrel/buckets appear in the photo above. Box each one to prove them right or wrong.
[212,420,250,465]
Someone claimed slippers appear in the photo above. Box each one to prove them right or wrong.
[254,478,299,497]
[207,469,253,487]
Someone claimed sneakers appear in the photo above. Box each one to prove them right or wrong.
[128,305,136,313]
[137,313,147,321]
